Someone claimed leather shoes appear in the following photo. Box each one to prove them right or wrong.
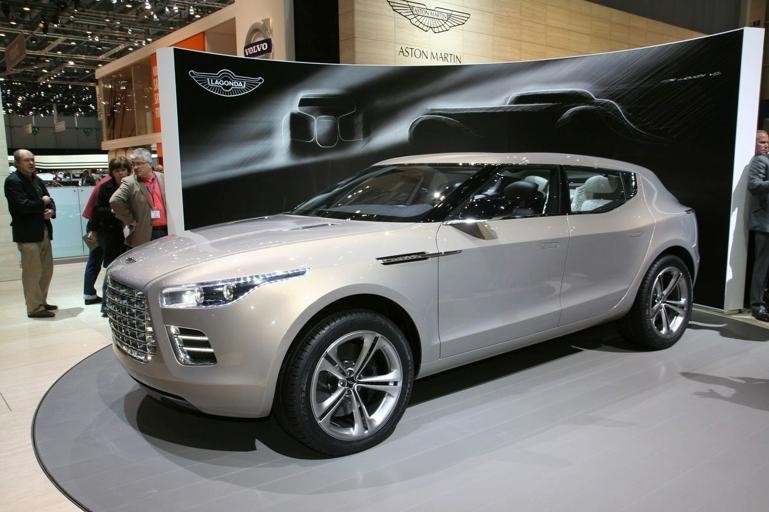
[45,304,57,311]
[27,310,53,317]
[85,297,104,305]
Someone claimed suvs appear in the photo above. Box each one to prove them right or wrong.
[103,152,701,457]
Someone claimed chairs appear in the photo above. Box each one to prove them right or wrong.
[524,176,550,214]
[582,174,625,211]
[503,181,547,215]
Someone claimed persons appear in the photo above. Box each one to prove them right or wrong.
[50,169,96,186]
[755,130,769,156]
[154,164,164,173]
[90,156,132,268]
[4,149,57,317]
[109,148,168,253]
[747,155,769,322]
[83,175,111,305]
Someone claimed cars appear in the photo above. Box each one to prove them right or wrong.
[53,170,84,183]
